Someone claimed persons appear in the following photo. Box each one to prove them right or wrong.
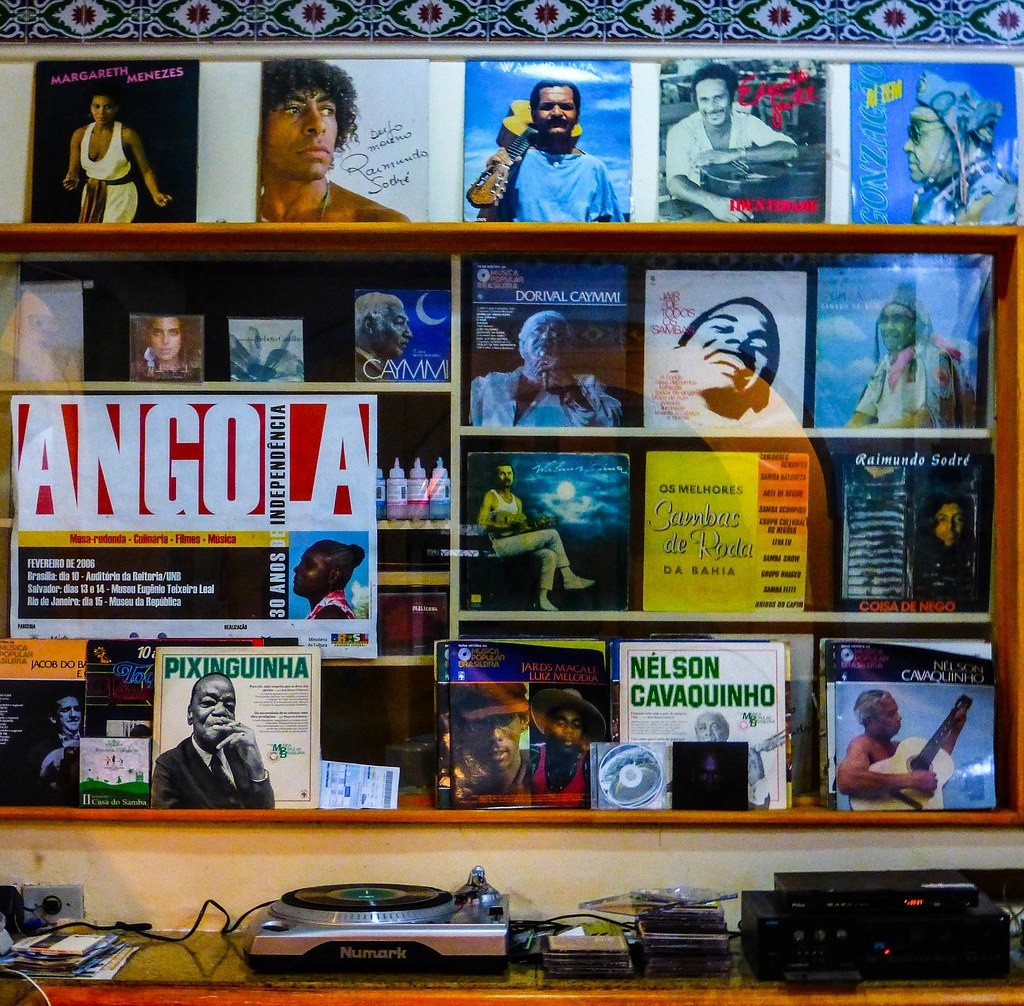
[290,539,365,620]
[925,496,979,602]
[263,59,414,223]
[471,308,624,426]
[454,682,536,813]
[152,671,277,810]
[475,463,601,612]
[476,74,626,222]
[834,687,975,809]
[356,292,414,381]
[62,90,173,223]
[897,80,1018,223]
[663,58,802,222]
[655,294,783,425]
[845,296,959,428]
[688,710,772,811]
[141,315,203,383]
[526,684,609,809]
[36,693,84,805]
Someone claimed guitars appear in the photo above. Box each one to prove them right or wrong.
[849,694,973,811]
[699,140,826,201]
[465,99,583,209]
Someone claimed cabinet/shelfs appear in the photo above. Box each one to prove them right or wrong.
[0,217,1024,1006]
